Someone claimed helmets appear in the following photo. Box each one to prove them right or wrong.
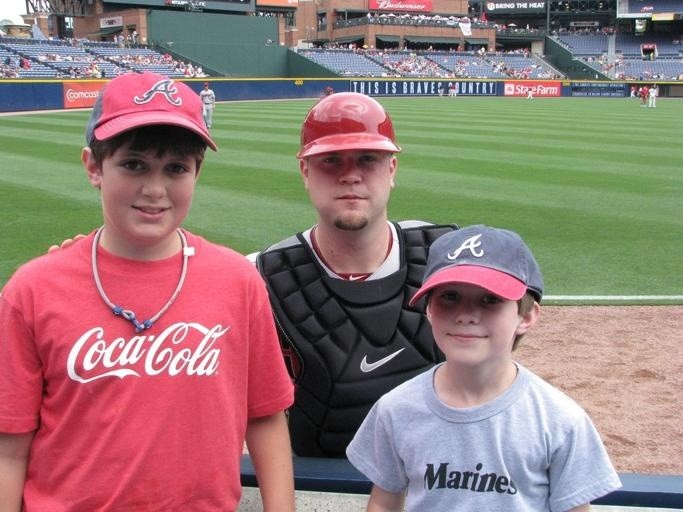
[296,91,402,160]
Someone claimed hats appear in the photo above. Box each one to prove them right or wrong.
[86,70,219,151]
[204,82,208,86]
[408,223,542,308]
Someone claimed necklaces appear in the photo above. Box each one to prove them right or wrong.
[90,223,189,333]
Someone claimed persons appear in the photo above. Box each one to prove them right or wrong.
[0,29,205,79]
[49,91,461,459]
[1,71,296,512]
[346,223,623,512]
[199,82,215,128]
